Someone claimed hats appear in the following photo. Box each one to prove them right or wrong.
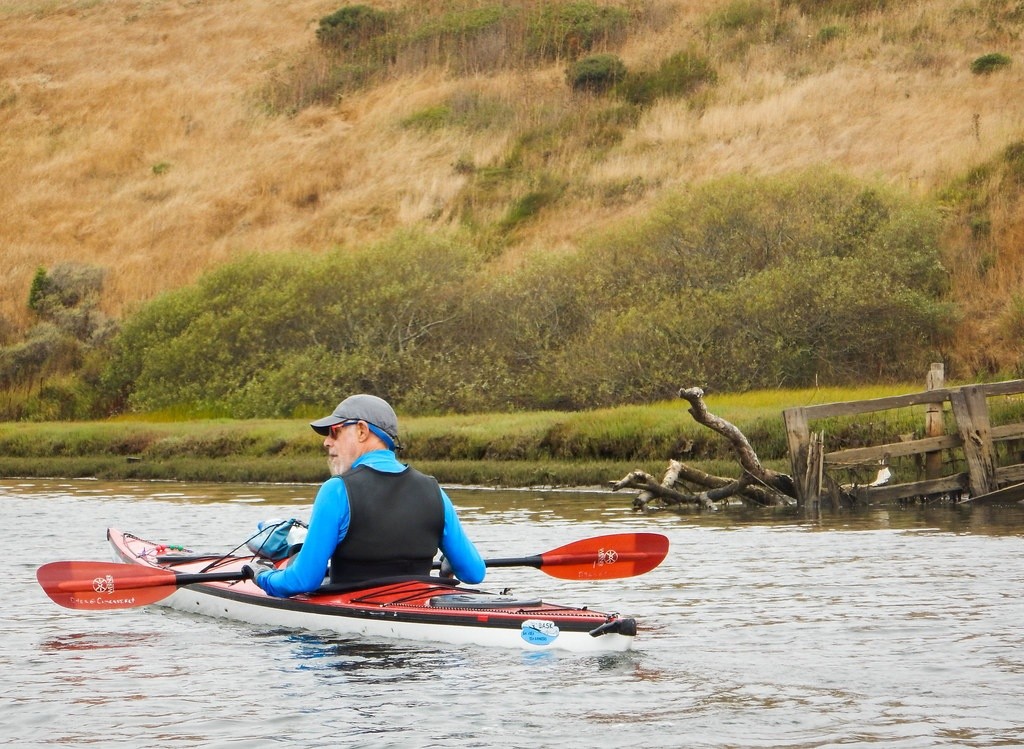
[310,394,398,440]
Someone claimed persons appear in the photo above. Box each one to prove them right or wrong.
[242,394,486,599]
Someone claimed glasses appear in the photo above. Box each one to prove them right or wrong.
[328,421,395,451]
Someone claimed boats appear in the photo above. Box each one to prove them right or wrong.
[105,526,639,654]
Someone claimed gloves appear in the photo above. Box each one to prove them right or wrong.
[439,553,454,579]
[241,559,275,584]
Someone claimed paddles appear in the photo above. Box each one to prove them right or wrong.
[36,532,671,610]
